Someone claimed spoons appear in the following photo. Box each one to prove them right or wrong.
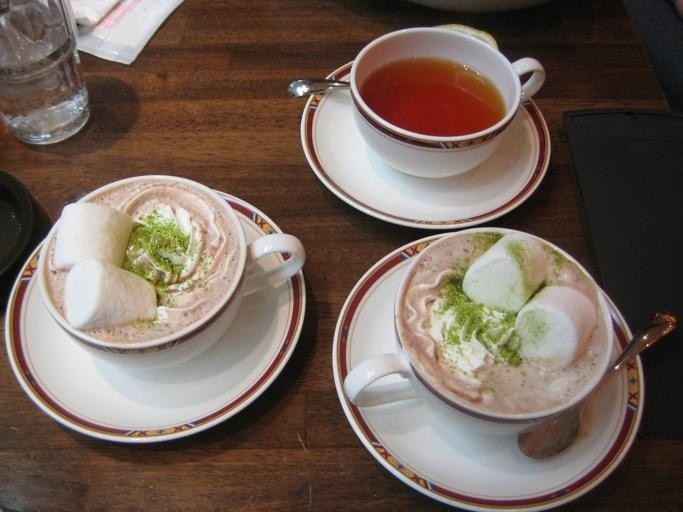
[515,309,677,461]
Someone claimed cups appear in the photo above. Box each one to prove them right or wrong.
[344,26,546,180]
[34,171,306,373]
[341,225,617,431]
[0,1,91,146]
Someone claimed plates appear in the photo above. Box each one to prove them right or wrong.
[327,224,646,510]
[3,181,312,447]
[298,58,553,230]
[0,173,34,275]
